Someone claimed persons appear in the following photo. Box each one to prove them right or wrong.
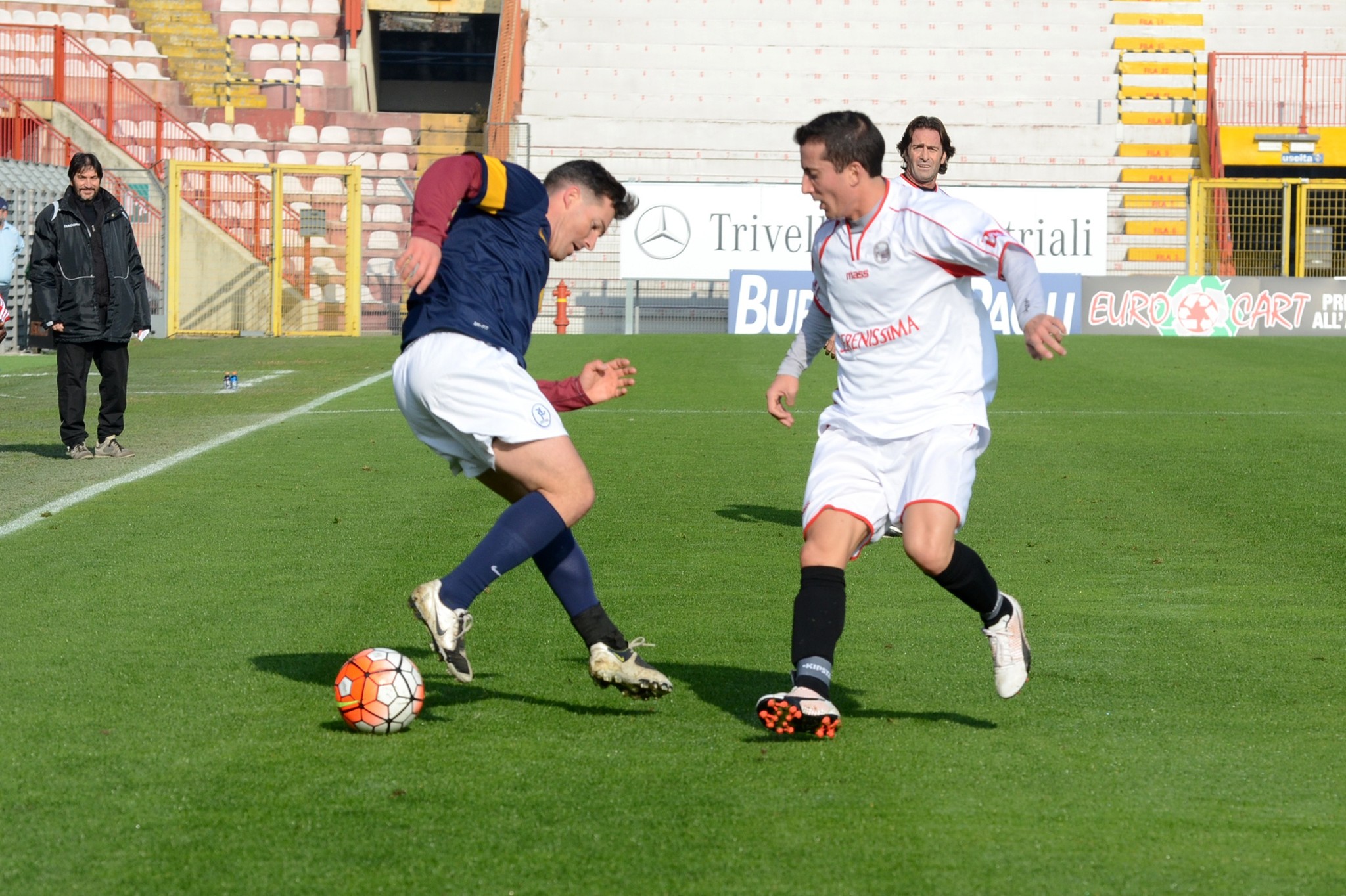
[391,150,674,703]
[0,197,23,343]
[27,153,151,460]
[753,111,1067,739]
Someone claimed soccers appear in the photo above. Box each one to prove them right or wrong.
[340,647,424,736]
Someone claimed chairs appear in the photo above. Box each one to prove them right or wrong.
[0,0,412,303]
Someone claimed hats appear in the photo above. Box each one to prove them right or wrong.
[0,198,7,210]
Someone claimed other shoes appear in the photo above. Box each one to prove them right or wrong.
[884,522,903,537]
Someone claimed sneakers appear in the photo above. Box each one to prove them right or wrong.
[70,443,93,460]
[981,591,1032,700]
[589,638,673,700]
[409,578,473,684]
[95,434,135,459]
[756,675,842,742]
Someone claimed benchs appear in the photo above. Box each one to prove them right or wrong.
[506,0,1346,277]
